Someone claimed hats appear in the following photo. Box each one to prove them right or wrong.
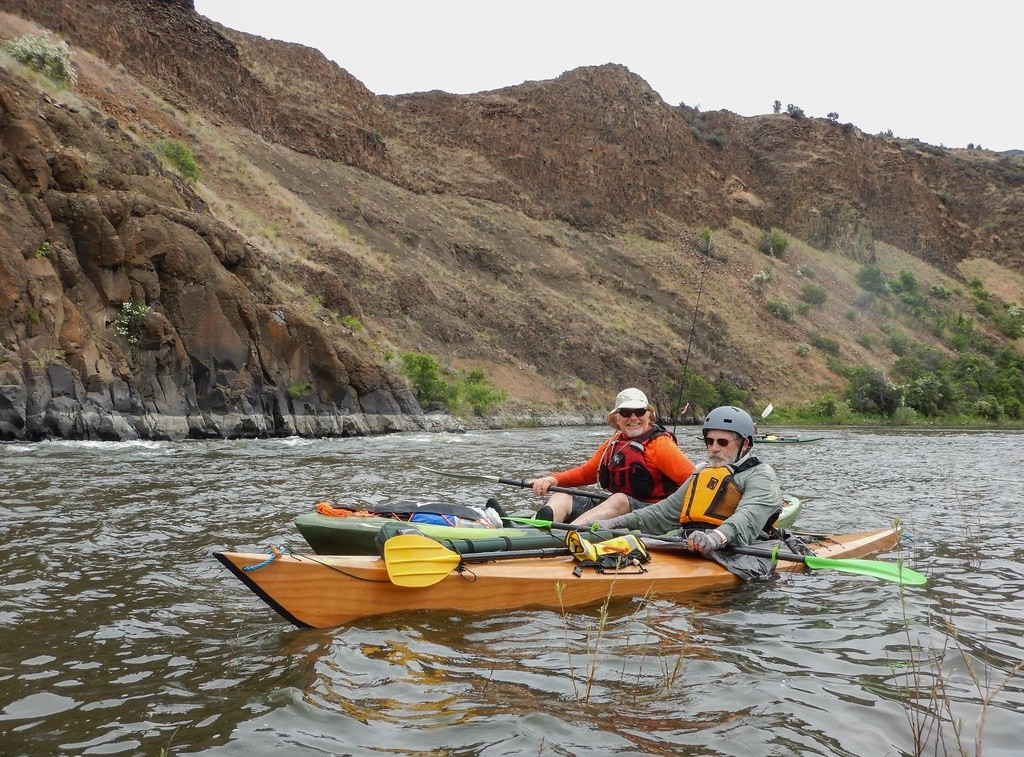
[607,388,656,431]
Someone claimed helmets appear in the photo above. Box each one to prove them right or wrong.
[702,406,754,447]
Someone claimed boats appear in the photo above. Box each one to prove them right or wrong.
[293,496,802,555]
[210,526,907,630]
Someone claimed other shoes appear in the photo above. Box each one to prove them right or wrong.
[486,498,517,528]
[532,506,553,528]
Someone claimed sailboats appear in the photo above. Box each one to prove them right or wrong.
[753,435,824,443]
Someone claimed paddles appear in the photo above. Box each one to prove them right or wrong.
[413,454,621,502]
[752,402,774,426]
[385,513,927,591]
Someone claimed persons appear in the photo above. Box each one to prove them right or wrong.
[581,406,782,555]
[486,388,698,529]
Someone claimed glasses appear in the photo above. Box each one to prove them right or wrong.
[619,408,646,417]
[704,437,739,447]
